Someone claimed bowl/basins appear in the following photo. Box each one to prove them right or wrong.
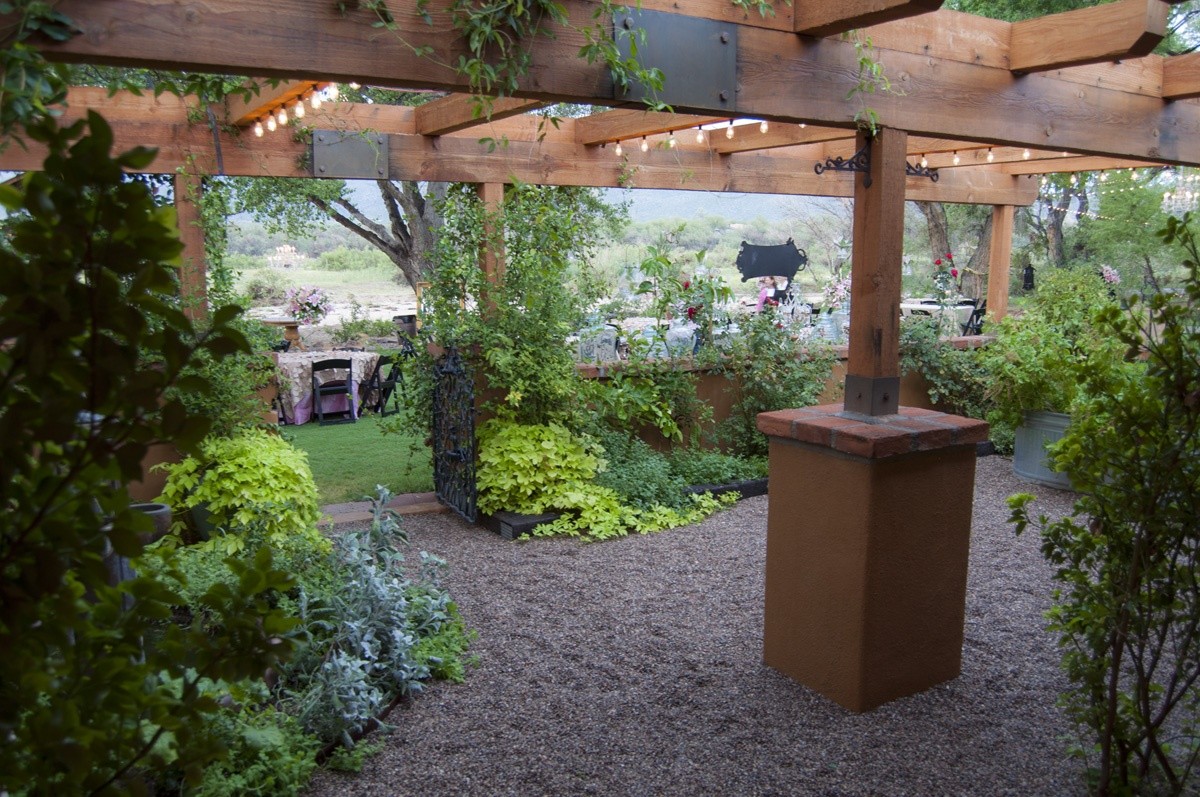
[106,503,173,557]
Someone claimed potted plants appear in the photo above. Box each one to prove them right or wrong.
[963,314,1140,495]
[162,347,292,541]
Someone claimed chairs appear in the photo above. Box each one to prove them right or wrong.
[397,330,418,366]
[332,347,365,352]
[254,366,288,426]
[312,356,355,425]
[268,340,291,352]
[357,353,409,417]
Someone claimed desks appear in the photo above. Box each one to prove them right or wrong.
[275,351,385,425]
[261,316,322,352]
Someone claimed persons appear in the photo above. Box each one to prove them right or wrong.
[755,273,796,315]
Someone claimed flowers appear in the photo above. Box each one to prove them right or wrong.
[283,283,334,326]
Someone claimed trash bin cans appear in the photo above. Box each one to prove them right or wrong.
[393,314,417,345]
